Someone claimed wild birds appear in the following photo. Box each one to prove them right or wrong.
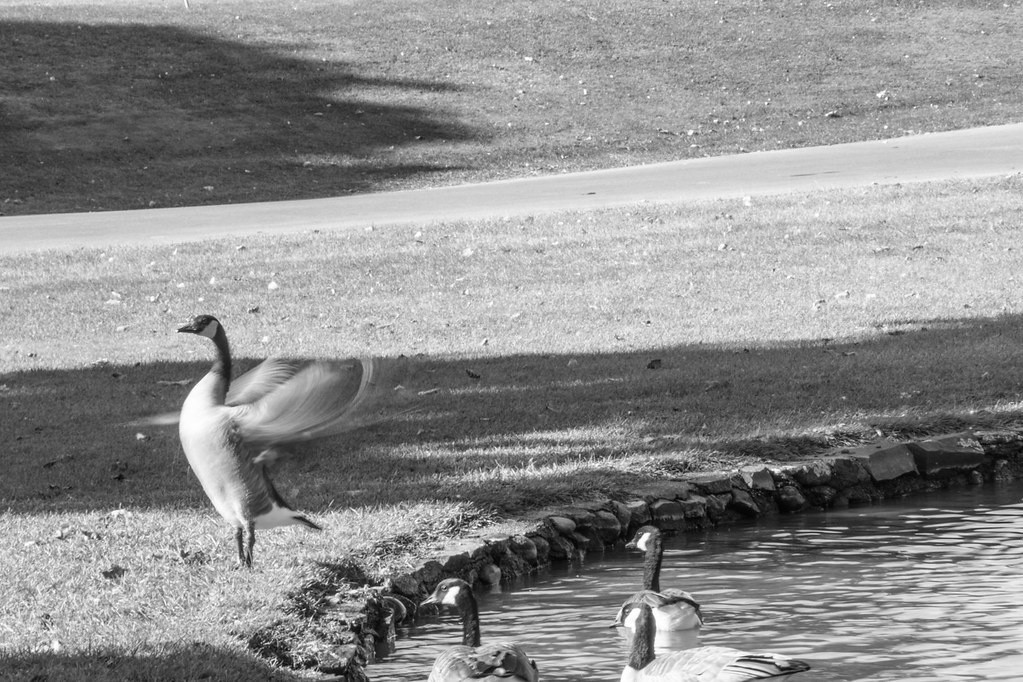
[416,577,542,682]
[614,525,705,633]
[609,602,811,682]
[174,314,375,573]
